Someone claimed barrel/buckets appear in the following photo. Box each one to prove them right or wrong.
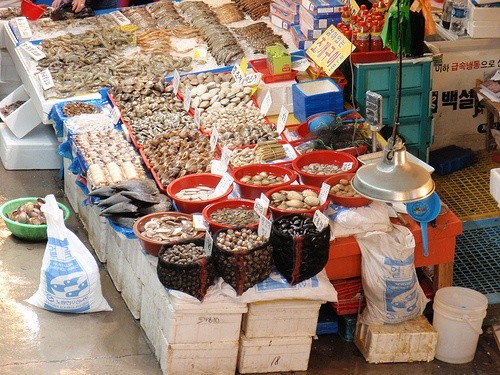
[433,286,488,364]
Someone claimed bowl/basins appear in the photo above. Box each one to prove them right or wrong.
[167,173,234,215]
[133,212,206,256]
[0,197,70,241]
[292,150,360,188]
[231,164,297,200]
[263,185,332,218]
[321,173,372,207]
[202,198,270,235]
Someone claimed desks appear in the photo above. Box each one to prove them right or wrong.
[317,193,463,294]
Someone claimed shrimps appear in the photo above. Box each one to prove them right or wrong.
[33,0,289,101]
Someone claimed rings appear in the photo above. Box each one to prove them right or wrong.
[80,4,84,7]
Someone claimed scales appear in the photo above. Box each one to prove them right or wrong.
[357,91,435,175]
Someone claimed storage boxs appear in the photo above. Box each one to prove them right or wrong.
[1,1,500,375]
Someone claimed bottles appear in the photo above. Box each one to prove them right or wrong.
[336,0,389,52]
[442,0,468,36]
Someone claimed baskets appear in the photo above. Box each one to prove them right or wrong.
[248,56,299,84]
[0,197,71,240]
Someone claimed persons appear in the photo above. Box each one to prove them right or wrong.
[53,0,132,13]
